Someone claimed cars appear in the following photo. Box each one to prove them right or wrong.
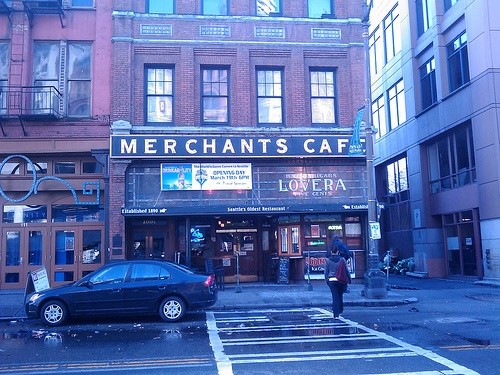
[25,258,219,327]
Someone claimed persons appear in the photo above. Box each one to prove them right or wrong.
[324,230,352,321]
[202,234,216,275]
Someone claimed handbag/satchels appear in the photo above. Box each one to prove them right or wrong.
[337,262,351,283]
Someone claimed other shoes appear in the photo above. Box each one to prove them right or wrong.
[335,316,343,319]
